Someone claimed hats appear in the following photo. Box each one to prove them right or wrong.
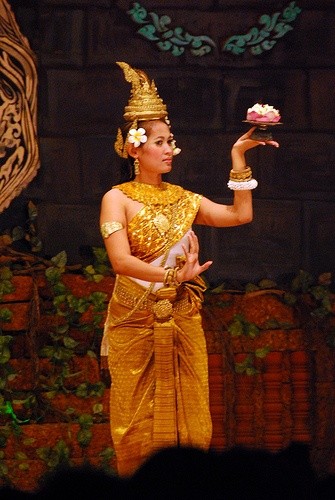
[114,62,181,158]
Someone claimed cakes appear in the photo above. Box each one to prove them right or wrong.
[246,103,281,123]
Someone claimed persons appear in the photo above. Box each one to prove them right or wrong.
[100,60,279,480]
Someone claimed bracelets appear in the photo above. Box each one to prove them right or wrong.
[168,269,178,288]
[230,171,252,179]
[174,267,182,285]
[231,167,251,173]
[229,175,252,182]
[227,179,260,191]
[163,266,172,288]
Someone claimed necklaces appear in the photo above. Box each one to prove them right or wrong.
[112,181,185,205]
